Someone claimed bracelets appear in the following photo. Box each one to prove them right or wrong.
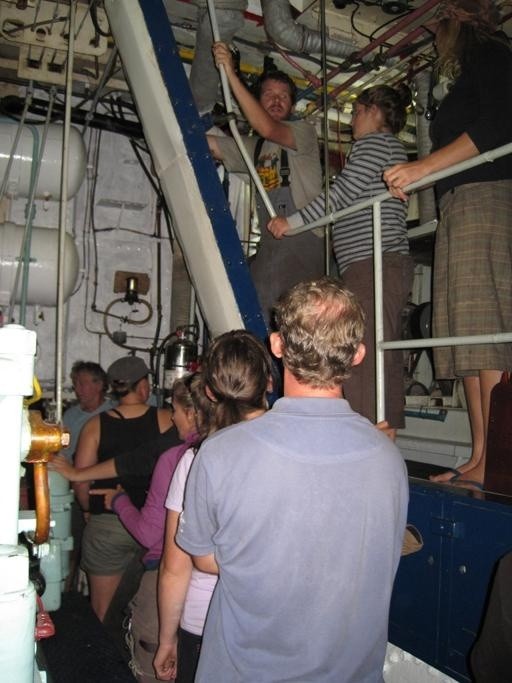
[83,509,90,514]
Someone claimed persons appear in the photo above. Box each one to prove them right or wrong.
[58,362,116,606]
[267,82,413,430]
[381,0,512,492]
[72,357,174,630]
[89,372,219,683]
[205,40,326,333]
[151,328,280,682]
[175,277,409,682]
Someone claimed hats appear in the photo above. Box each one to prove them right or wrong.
[108,357,156,392]
